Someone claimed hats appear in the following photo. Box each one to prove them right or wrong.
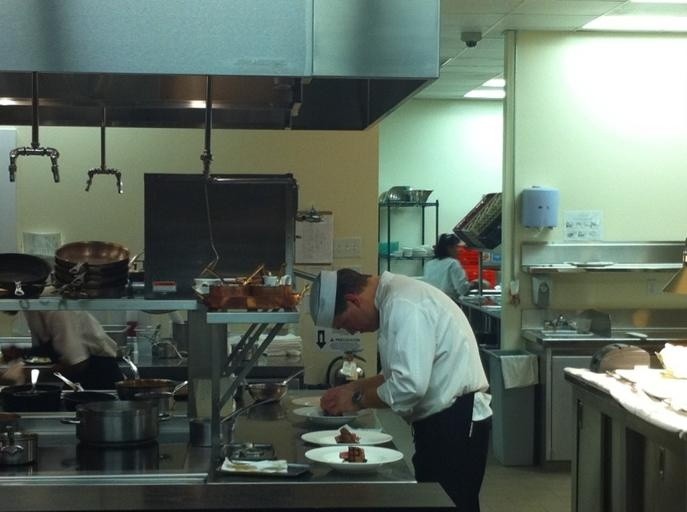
[310,271,337,328]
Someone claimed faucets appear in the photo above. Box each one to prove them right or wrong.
[556,315,565,328]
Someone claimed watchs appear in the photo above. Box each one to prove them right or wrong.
[352,381,366,410]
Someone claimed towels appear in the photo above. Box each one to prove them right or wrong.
[472,391,493,422]
[500,355,540,390]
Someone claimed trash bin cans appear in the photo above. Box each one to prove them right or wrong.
[484,349,539,467]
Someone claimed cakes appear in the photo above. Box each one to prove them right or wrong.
[334,423,362,443]
[339,444,367,464]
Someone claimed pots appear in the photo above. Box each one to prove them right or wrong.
[243,361,316,403]
[0,239,134,300]
[379,185,432,204]
[0,353,186,467]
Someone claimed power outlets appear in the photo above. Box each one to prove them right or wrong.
[334,238,362,258]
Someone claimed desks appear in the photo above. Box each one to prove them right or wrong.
[561,364,687,512]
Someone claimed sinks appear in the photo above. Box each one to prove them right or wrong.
[540,329,595,337]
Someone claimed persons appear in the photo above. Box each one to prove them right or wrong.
[424,234,471,310]
[0,285,123,367]
[310,267,494,512]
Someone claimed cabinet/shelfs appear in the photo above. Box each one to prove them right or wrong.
[378,199,439,276]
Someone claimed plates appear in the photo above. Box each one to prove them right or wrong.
[299,428,395,446]
[292,403,366,427]
[302,448,404,473]
[289,395,329,408]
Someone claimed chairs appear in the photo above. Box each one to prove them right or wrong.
[589,344,651,371]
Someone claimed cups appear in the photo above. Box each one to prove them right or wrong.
[189,267,291,293]
[377,240,435,259]
